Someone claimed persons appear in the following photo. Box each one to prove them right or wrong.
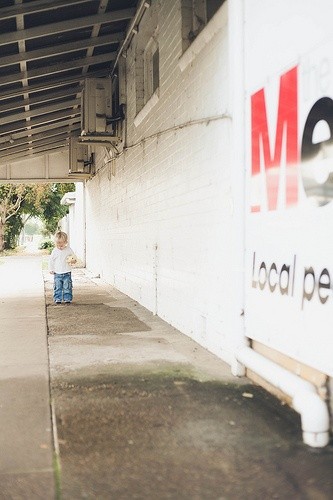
[48,232,78,304]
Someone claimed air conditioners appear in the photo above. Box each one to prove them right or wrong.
[81,77,114,137]
[68,135,89,173]
